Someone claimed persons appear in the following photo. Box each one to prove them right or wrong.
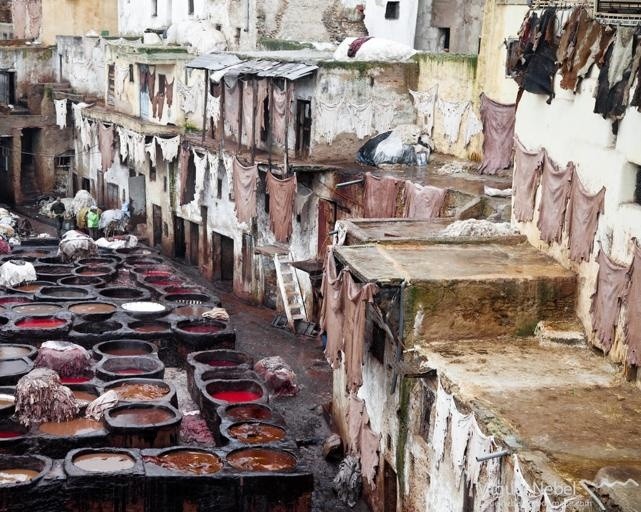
[49,197,67,239]
[86,205,103,241]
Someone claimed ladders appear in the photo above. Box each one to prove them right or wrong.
[274,251,307,336]
